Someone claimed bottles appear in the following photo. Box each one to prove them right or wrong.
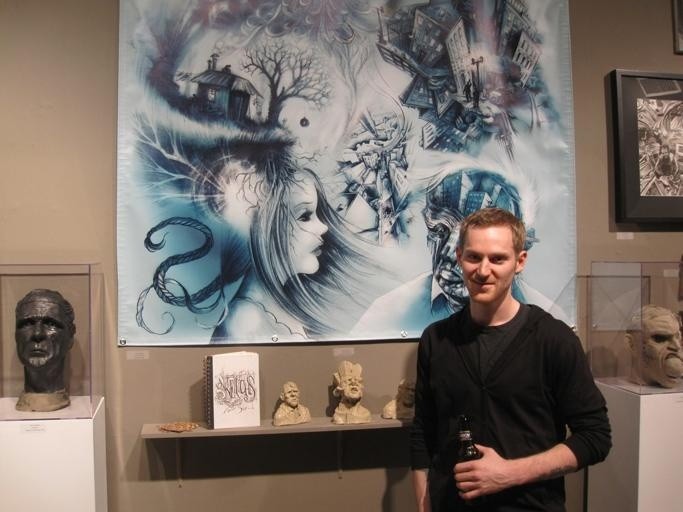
[455,413,484,508]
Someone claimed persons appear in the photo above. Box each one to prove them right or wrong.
[274,381,312,427]
[331,360,372,424]
[624,305,682,390]
[654,144,680,190]
[14,288,76,413]
[382,378,415,420]
[406,207,612,511]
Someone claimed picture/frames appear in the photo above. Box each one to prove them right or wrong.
[672,0,683,54]
[610,68,683,223]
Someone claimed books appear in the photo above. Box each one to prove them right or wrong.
[202,351,261,429]
